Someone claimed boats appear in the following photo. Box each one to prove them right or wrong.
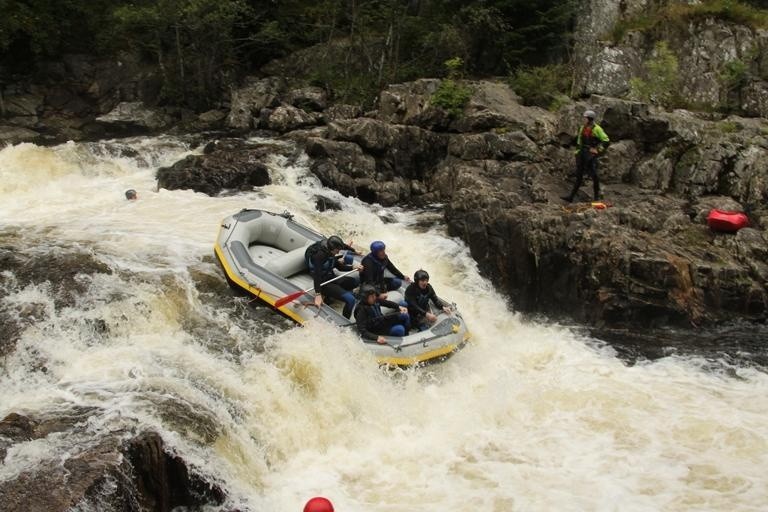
[214,207,470,373]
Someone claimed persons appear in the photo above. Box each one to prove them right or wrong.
[404,270,450,328]
[307,236,363,320]
[353,285,409,345]
[359,241,411,293]
[560,109,610,203]
[125,189,137,201]
[303,496,334,512]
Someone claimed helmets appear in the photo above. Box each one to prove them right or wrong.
[327,236,345,250]
[414,269,429,281]
[359,283,377,298]
[369,241,385,257]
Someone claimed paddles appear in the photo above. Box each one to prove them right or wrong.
[275,268,358,307]
